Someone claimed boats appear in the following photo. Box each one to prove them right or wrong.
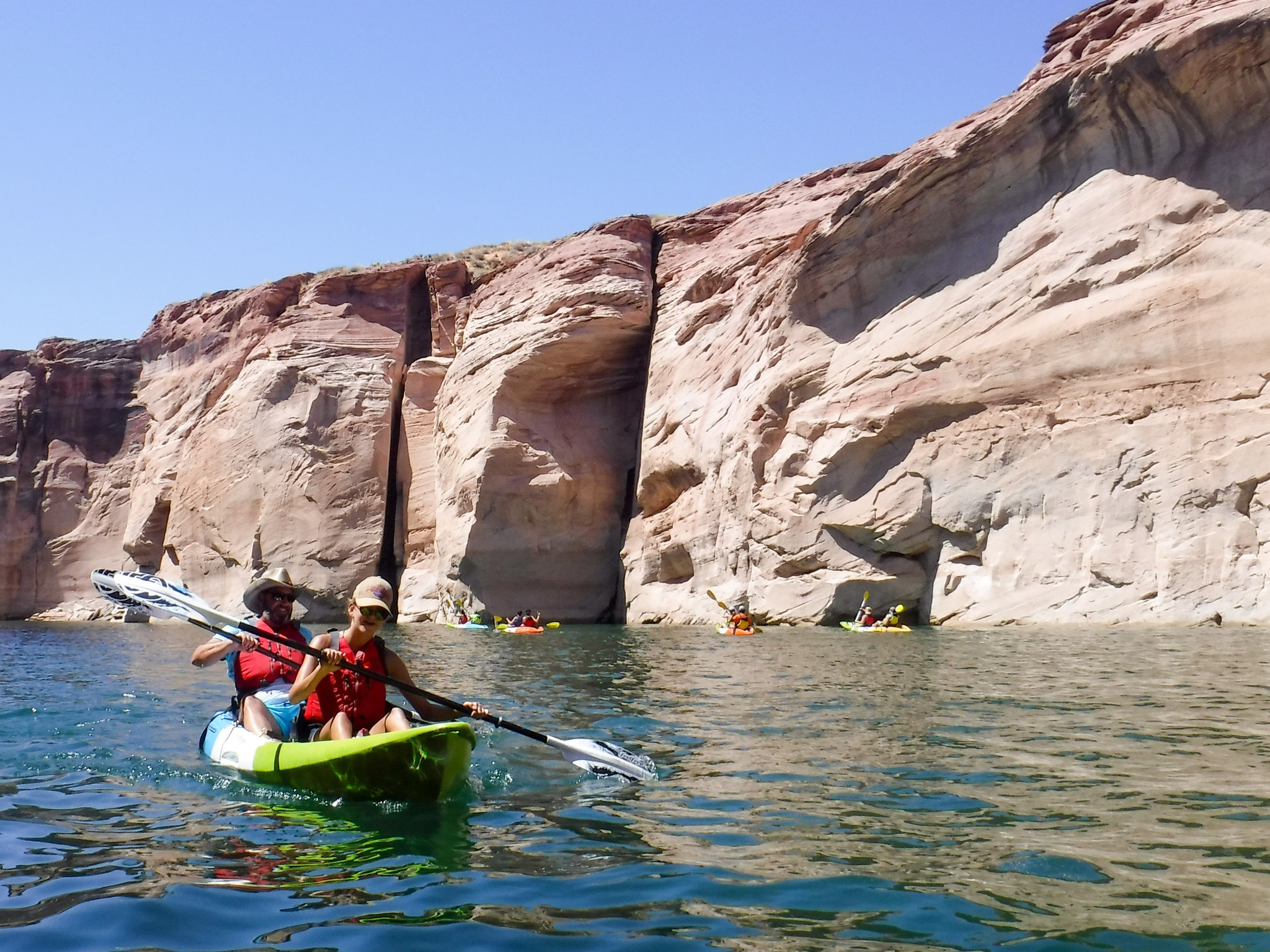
[839,620,913,634]
[446,621,489,630]
[502,627,544,634]
[197,694,477,803]
[715,620,754,635]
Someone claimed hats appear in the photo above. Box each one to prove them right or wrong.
[865,606,872,615]
[729,606,735,610]
[352,576,393,616]
[739,605,745,611]
[890,606,895,611]
[244,567,307,614]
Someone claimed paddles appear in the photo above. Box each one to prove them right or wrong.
[851,591,869,631]
[496,622,560,631]
[718,602,763,633]
[494,616,505,622]
[114,571,659,784]
[873,604,904,631]
[90,568,458,724]
[707,590,730,615]
[445,599,453,610]
[456,598,470,615]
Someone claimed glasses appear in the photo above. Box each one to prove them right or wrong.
[354,602,389,621]
[264,589,296,603]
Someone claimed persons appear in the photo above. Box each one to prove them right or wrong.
[287,576,489,741]
[453,609,482,624]
[191,567,313,739]
[505,609,540,627]
[722,605,751,628]
[854,606,873,627]
[872,607,899,627]
